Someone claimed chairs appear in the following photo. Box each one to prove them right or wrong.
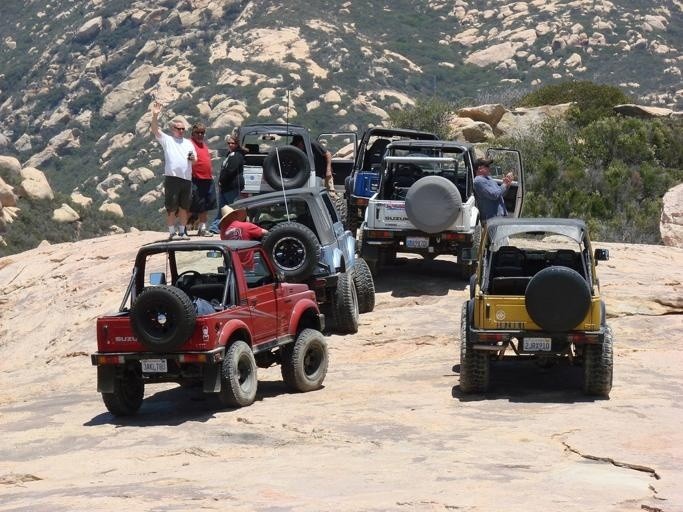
[490,246,576,295]
[190,284,229,305]
[245,144,259,153]
[397,165,418,187]
[367,139,389,163]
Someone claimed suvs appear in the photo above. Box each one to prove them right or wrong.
[222,186,375,336]
[89,236,330,417]
[341,127,439,233]
[457,213,614,398]
[217,124,360,203]
[355,137,527,284]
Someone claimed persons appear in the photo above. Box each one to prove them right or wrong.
[290,133,333,192]
[218,205,269,282]
[152,103,198,240]
[472,158,514,226]
[218,134,244,208]
[189,123,216,237]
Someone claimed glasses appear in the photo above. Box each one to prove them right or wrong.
[174,127,185,130]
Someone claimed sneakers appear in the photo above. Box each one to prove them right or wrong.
[169,233,190,239]
[198,230,213,237]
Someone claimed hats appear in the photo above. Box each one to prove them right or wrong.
[474,158,493,170]
[218,204,247,230]
[290,136,302,145]
